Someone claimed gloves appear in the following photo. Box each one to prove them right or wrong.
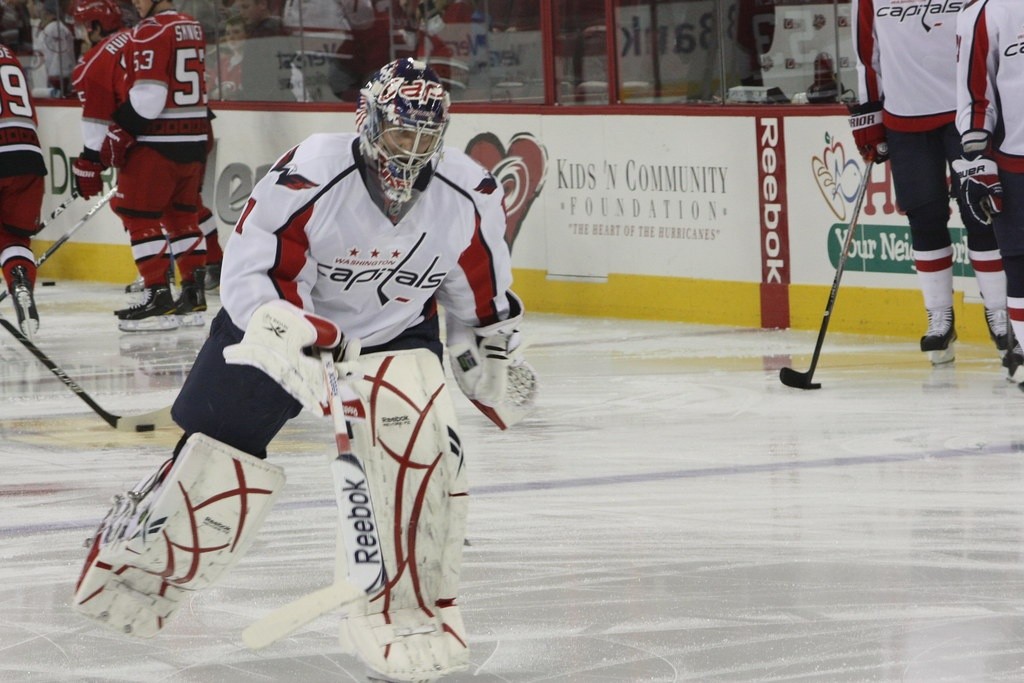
[100,122,136,167]
[222,299,369,423]
[444,288,540,430]
[73,156,109,201]
[950,152,1004,227]
[848,101,890,165]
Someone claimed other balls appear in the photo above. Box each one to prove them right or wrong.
[806,382,822,390]
[135,423,155,432]
[42,280,55,286]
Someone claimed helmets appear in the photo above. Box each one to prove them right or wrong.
[68,0,123,38]
[354,57,450,201]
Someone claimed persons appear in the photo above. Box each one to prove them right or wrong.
[205,0,776,102]
[75,60,534,683]
[101,0,210,331]
[0,44,48,341]
[955,0,1024,392]
[67,0,222,303]
[848,0,1017,364]
[27,0,75,97]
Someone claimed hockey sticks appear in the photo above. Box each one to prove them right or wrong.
[238,346,385,655]
[0,184,117,303]
[0,314,183,433]
[778,148,877,391]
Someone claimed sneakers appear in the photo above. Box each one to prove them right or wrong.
[9,264,40,337]
[205,259,222,291]
[983,306,1015,350]
[919,305,958,364]
[112,267,207,332]
